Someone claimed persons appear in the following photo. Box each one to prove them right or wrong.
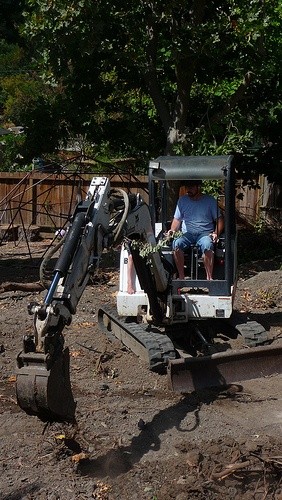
[167,180,224,281]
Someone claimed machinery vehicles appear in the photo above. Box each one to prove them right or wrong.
[16,156,269,424]
